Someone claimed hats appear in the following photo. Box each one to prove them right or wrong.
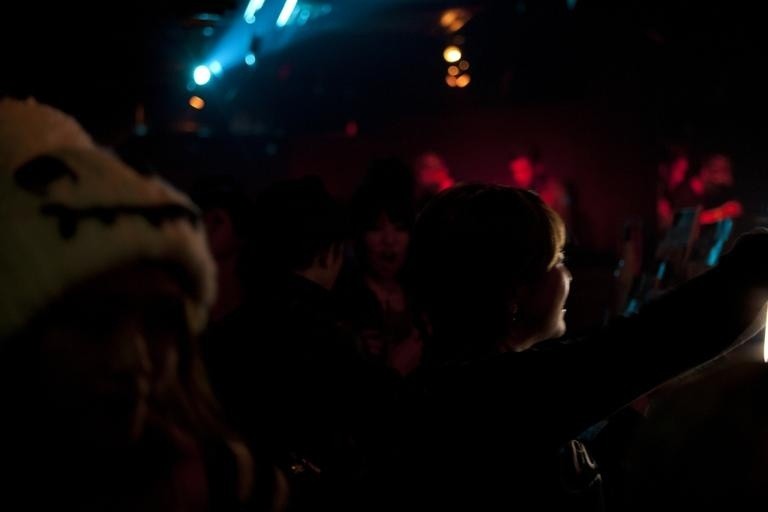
[0,103,217,333]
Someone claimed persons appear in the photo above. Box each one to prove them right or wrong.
[0,97,768,512]
